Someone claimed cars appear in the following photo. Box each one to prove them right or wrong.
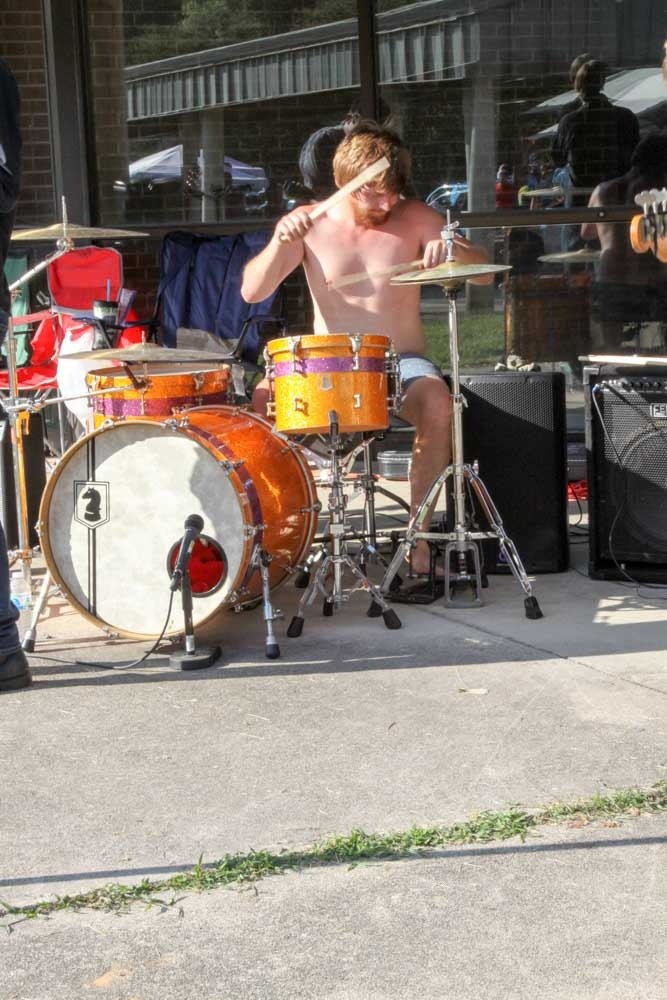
[425,121,601,282]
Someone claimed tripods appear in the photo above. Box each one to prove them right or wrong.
[285,290,543,637]
[4,238,76,611]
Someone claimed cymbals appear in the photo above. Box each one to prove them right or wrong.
[10,223,150,240]
[538,249,601,263]
[55,342,235,361]
[520,186,592,196]
[390,261,514,288]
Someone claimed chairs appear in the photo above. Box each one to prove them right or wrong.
[70,229,290,401]
[0,246,148,455]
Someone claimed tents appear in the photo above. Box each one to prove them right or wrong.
[129,145,270,220]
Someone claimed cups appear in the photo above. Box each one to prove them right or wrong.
[93,300,119,349]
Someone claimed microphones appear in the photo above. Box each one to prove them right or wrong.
[168,515,203,591]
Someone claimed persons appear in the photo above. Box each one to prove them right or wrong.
[300,125,346,195]
[526,40,667,372]
[496,152,550,235]
[0,58,34,694]
[496,169,515,207]
[242,109,494,604]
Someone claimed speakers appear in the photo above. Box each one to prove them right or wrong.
[442,373,570,575]
[585,364,667,586]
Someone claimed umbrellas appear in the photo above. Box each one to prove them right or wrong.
[520,68,667,140]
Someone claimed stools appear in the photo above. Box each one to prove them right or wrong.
[356,414,416,577]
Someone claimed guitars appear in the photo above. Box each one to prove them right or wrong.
[629,188,667,264]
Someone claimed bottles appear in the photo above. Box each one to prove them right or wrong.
[10,572,33,644]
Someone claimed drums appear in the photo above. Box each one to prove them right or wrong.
[37,405,322,640]
[85,362,232,430]
[503,272,591,367]
[266,332,390,434]
